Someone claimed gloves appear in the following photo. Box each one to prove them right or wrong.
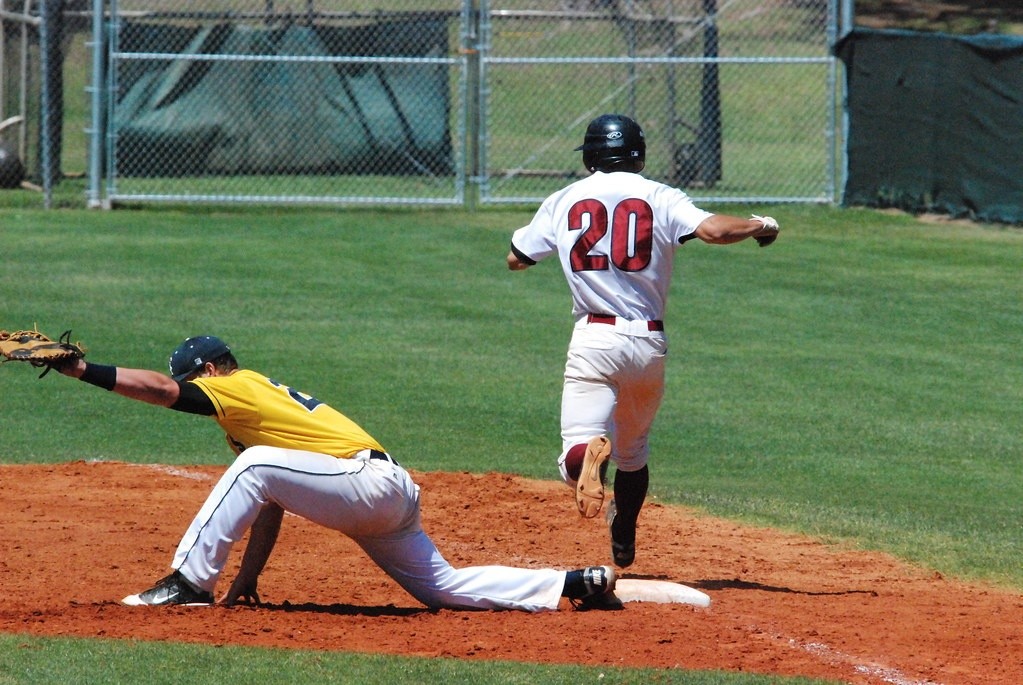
[748,213,781,247]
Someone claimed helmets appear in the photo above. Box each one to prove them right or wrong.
[573,114,646,173]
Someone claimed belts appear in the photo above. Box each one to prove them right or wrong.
[370,448,398,466]
[587,312,664,331]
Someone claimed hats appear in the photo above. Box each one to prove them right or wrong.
[168,336,231,382]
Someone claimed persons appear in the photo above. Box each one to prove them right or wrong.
[505,114,780,569]
[0,323,626,612]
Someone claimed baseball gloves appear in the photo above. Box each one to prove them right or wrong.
[0,320,88,380]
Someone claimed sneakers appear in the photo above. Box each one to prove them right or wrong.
[576,436,612,519]
[605,497,635,568]
[120,570,215,606]
[568,565,615,611]
